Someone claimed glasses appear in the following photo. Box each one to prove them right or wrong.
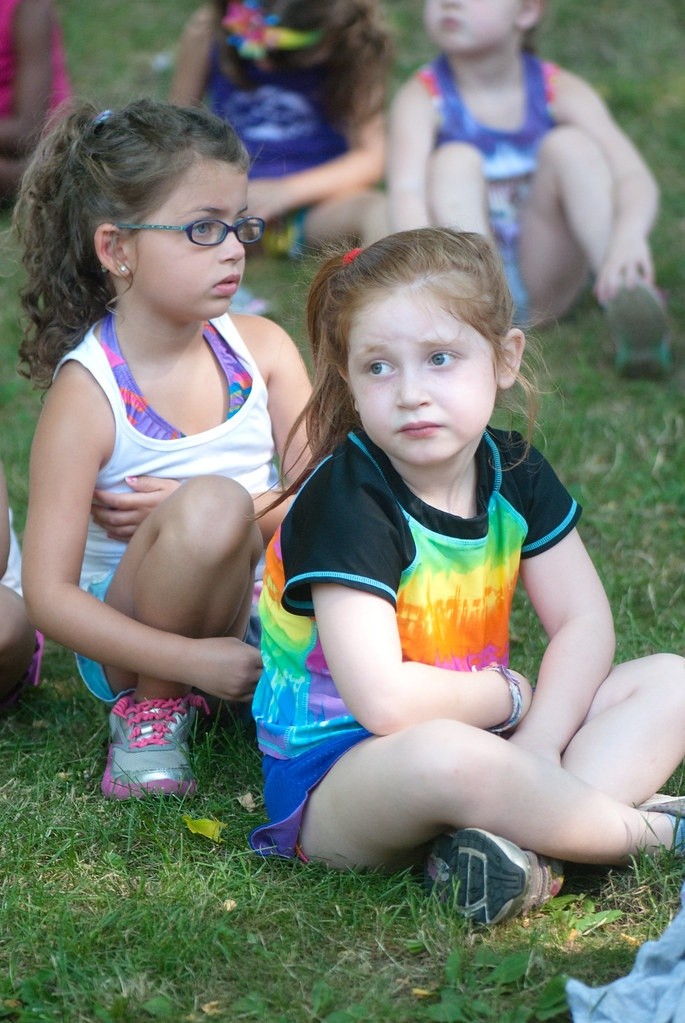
[115,215,266,246]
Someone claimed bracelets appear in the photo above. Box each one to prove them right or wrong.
[467,662,522,736]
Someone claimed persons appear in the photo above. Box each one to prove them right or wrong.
[253,226,685,932]
[0,465,42,726]
[161,0,667,380]
[0,0,72,211]
[15,96,324,799]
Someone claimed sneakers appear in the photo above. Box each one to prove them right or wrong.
[639,794,685,859]
[101,693,211,802]
[424,827,563,929]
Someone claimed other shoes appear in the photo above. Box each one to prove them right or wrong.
[611,278,673,379]
[233,289,274,315]
